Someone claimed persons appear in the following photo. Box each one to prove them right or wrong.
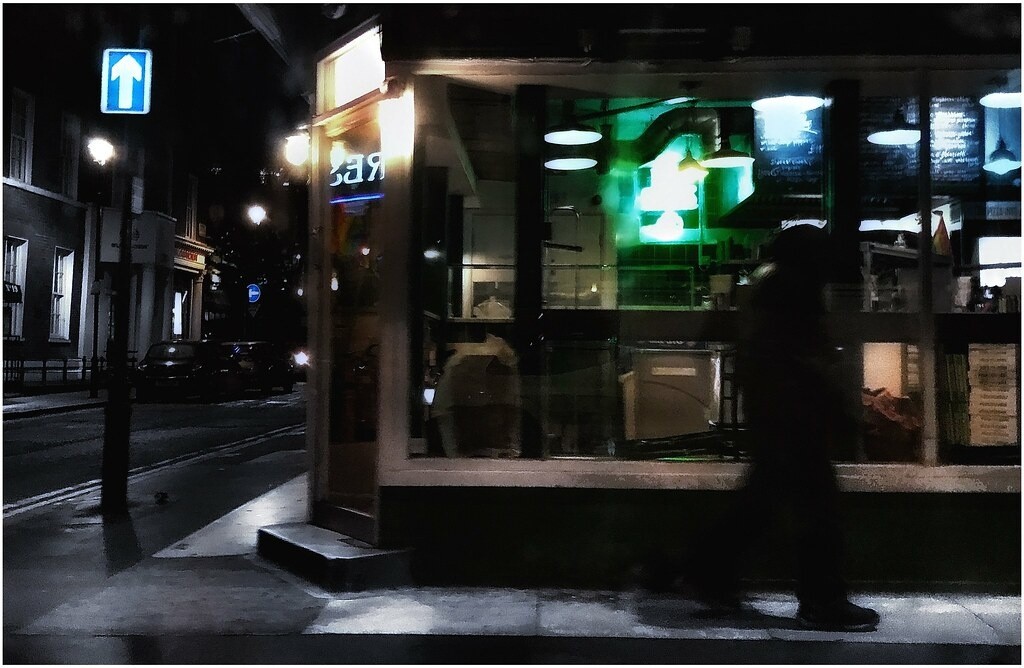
[682,223,879,632]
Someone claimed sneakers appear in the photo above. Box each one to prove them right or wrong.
[691,593,769,621]
[795,599,880,631]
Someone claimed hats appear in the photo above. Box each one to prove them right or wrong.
[771,224,840,260]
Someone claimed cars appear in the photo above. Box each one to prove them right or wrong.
[136,337,309,402]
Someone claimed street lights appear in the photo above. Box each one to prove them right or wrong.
[85,134,117,399]
